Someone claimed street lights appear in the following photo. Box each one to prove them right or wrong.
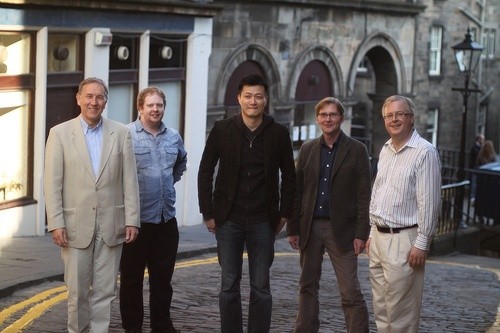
[450,26,483,229]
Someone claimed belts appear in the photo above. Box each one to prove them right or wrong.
[376,224,418,233]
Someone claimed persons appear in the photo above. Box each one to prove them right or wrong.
[44,77,141,333]
[366,95,442,333]
[287,97,372,333]
[470,135,497,205]
[197,75,296,333]
[119,86,186,333]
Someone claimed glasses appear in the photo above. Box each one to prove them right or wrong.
[383,112,413,120]
[317,112,341,118]
